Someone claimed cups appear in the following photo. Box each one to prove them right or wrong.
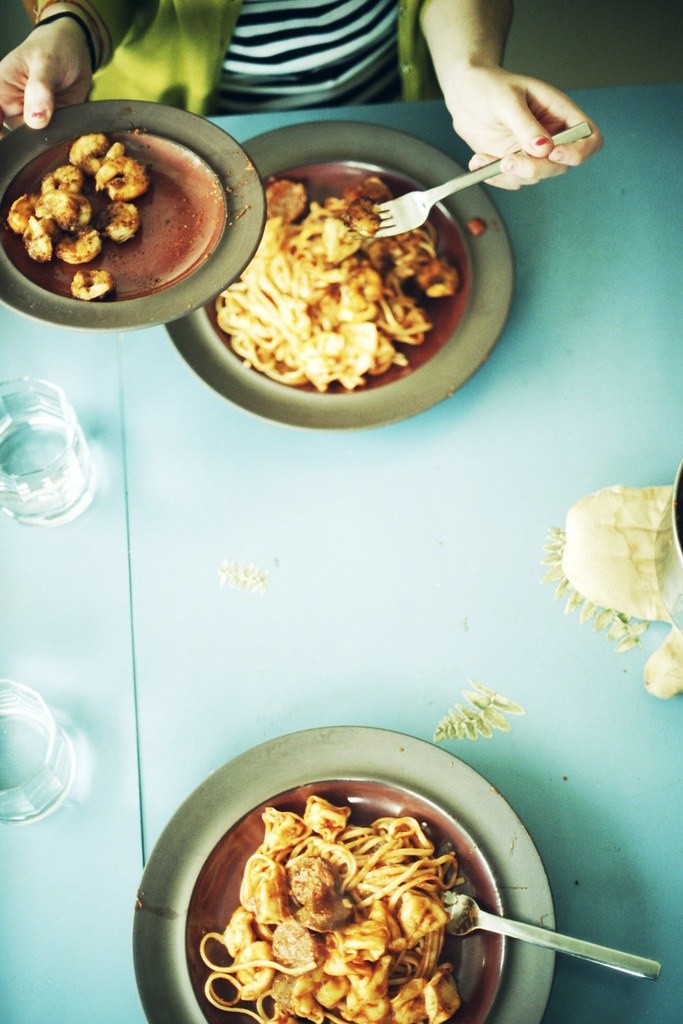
[0,376,99,529]
[0,679,77,827]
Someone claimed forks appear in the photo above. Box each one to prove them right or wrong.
[369,121,591,237]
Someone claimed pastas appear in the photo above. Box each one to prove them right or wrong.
[216,197,437,393]
[200,819,461,1024]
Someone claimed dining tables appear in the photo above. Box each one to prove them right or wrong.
[0,85,683,1024]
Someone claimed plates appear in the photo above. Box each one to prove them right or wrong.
[163,118,516,434]
[132,726,557,1024]
[0,99,267,333]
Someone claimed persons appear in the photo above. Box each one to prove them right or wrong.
[0,2,604,190]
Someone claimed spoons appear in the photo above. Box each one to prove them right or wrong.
[443,891,661,981]
